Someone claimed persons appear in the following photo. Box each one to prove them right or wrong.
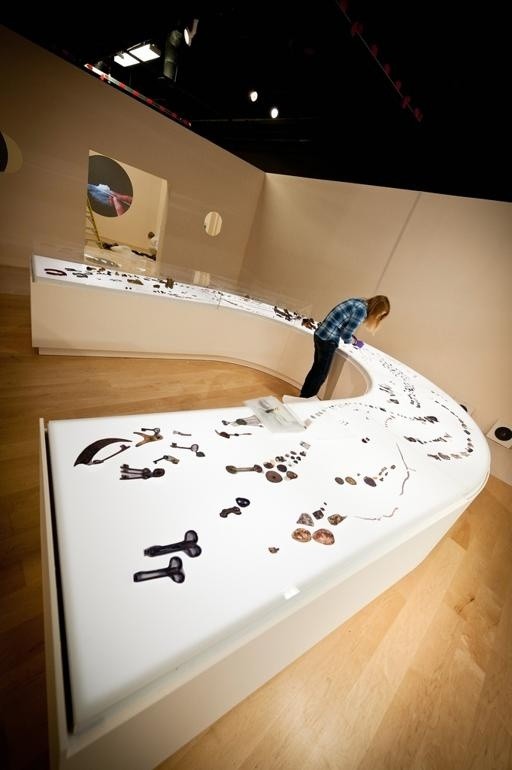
[298,294,390,398]
[146,231,159,256]
[108,192,133,217]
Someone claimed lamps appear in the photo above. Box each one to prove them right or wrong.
[112,19,200,68]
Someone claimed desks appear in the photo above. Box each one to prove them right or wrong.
[29,254,492,770]
